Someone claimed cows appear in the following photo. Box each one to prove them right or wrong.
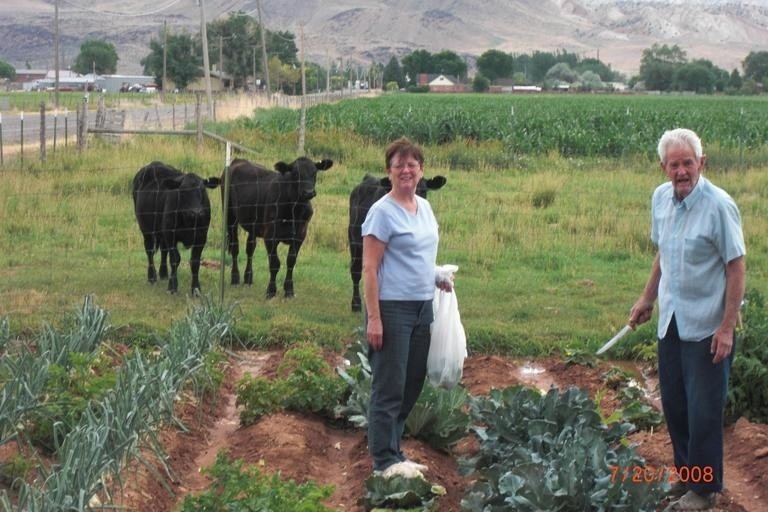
[221,156,334,300]
[347,173,447,312]
[131,160,221,299]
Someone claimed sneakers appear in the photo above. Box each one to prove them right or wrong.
[662,477,717,511]
[373,459,428,480]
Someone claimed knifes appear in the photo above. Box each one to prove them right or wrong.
[594,320,638,356]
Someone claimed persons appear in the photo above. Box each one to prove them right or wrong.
[630,129,746,510]
[362,135,456,476]
